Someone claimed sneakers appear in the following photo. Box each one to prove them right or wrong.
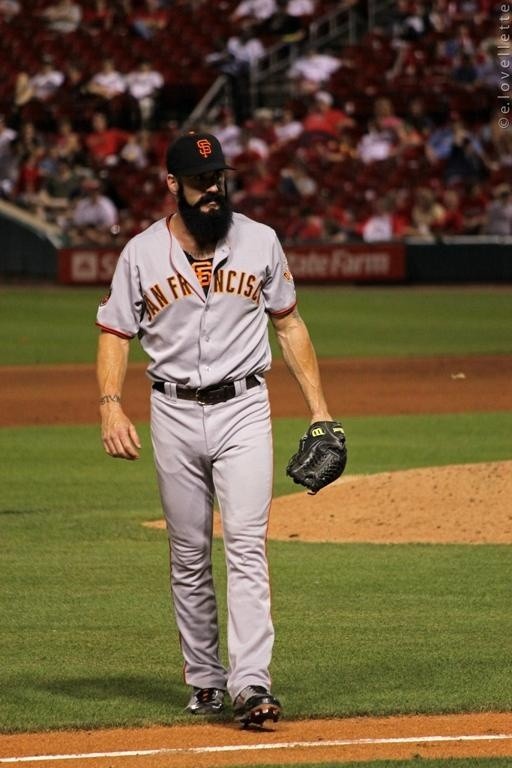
[189,685,226,716]
[232,684,281,727]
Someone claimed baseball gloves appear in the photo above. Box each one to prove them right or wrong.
[285,421,347,495]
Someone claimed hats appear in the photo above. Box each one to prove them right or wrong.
[165,130,240,178]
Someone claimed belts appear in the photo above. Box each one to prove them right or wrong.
[153,371,265,406]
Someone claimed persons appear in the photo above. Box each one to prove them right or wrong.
[1,2,510,248]
[93,128,347,727]
